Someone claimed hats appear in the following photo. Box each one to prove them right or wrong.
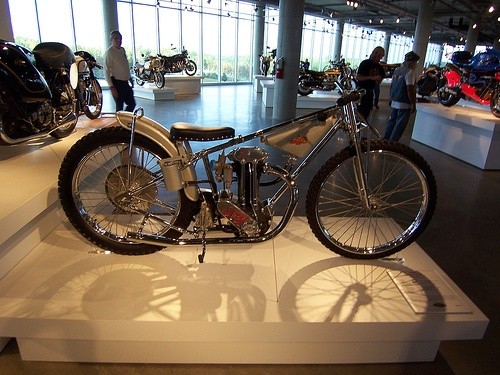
[405,51,420,62]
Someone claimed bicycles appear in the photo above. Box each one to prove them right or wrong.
[57,63,439,264]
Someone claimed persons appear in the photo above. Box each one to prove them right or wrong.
[383,51,420,142]
[355,46,385,121]
[303,59,310,69]
[103,31,136,112]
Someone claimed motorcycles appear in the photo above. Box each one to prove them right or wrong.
[134,46,198,89]
[0,39,103,146]
[417,44,500,118]
[258,46,358,96]
